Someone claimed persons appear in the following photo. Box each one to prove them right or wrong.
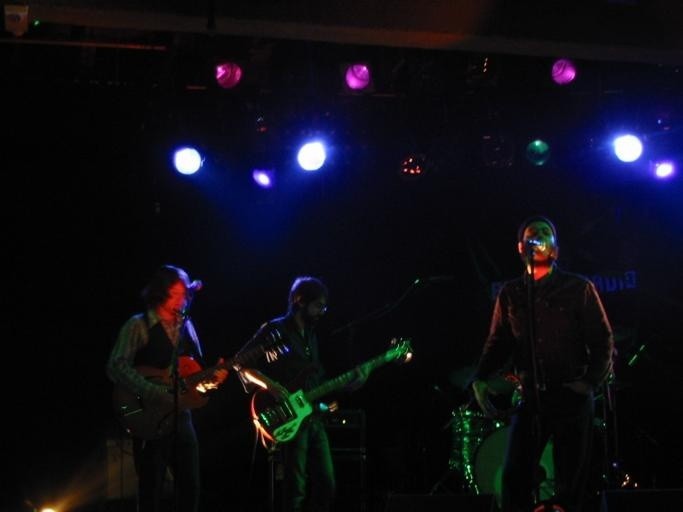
[107,266,229,512]
[462,216,614,512]
[235,275,367,512]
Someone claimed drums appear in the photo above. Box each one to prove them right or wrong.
[448,410,504,480]
[472,425,556,508]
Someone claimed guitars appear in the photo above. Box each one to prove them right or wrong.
[114,329,282,433]
[250,338,413,442]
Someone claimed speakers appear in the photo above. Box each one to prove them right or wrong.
[598,488,683,512]
[388,492,497,512]
[324,407,368,511]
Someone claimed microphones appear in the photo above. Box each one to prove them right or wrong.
[184,279,203,291]
[527,239,547,252]
